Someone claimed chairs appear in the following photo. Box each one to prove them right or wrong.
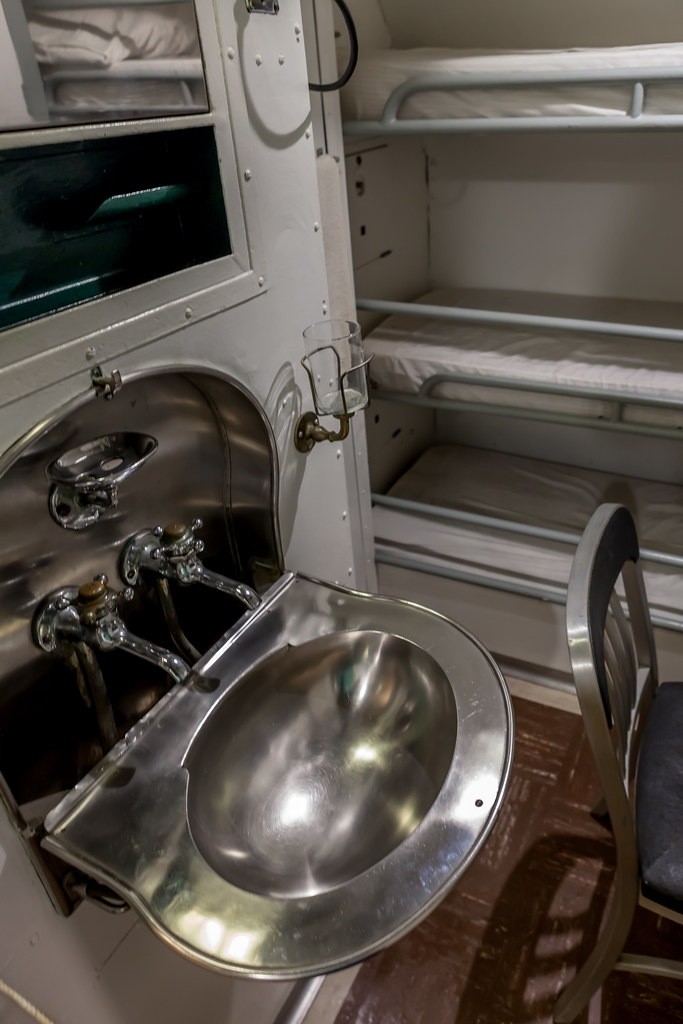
[550,502,683,1024]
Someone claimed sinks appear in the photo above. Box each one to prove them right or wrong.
[40,568,513,985]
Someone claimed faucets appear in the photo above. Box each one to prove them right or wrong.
[55,573,193,685]
[151,517,262,612]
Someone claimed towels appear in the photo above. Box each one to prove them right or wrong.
[22,0,210,124]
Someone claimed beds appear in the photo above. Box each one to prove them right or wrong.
[343,42,683,633]
